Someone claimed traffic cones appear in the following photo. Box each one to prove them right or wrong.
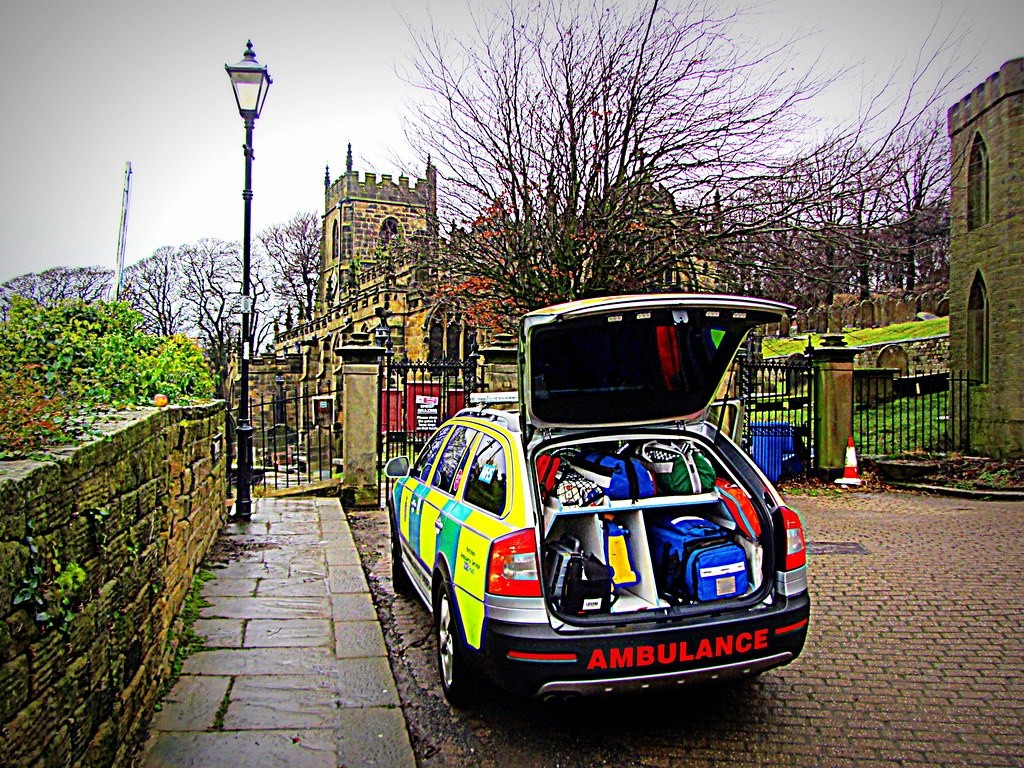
[834,437,863,485]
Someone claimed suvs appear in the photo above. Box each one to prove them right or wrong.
[384,293,811,717]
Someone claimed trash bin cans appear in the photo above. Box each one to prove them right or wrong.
[748,419,795,483]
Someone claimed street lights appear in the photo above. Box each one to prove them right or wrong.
[224,39,273,523]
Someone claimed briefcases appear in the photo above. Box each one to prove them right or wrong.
[544,534,579,597]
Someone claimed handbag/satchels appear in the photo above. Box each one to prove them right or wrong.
[536,439,717,506]
[648,515,748,602]
[561,552,620,615]
